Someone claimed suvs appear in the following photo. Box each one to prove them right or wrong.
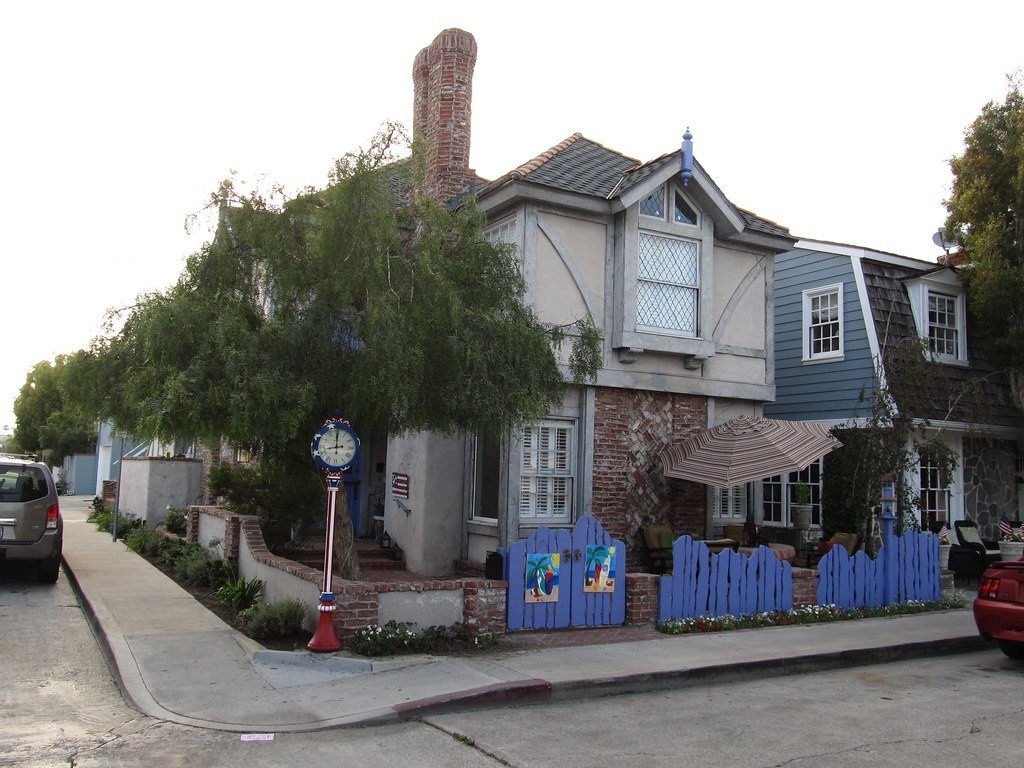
[0,451,64,585]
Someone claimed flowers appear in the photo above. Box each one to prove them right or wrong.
[1003,532,1024,544]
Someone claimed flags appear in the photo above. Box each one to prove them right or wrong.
[1019,522,1024,536]
[937,523,946,542]
[999,513,1013,535]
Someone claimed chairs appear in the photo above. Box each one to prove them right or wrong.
[807,532,858,569]
[645,523,759,572]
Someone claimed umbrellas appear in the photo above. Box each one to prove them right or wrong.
[656,415,844,543]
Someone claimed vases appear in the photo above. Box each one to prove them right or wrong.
[998,541,1024,561]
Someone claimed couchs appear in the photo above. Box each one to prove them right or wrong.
[954,520,1001,581]
[930,520,979,583]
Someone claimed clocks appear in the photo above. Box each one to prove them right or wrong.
[310,423,361,475]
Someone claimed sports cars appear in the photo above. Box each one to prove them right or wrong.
[973,550,1024,662]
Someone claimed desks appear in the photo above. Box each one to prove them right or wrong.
[736,541,795,566]
